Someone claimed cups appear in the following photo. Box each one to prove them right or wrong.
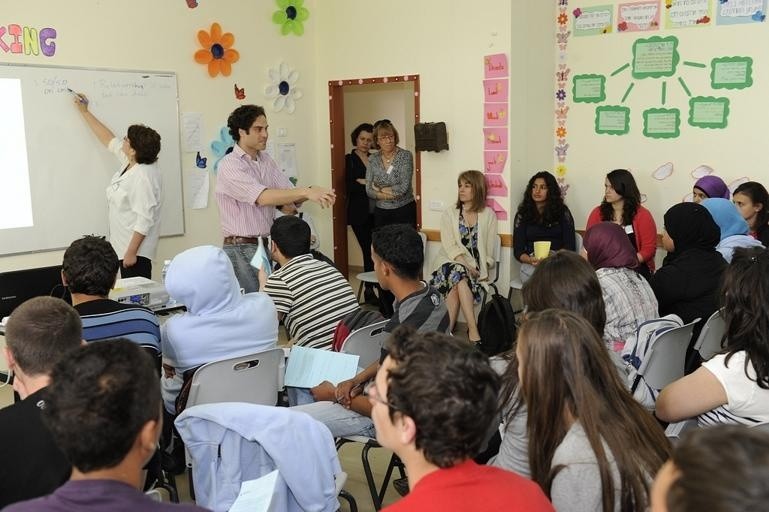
[534,241,551,258]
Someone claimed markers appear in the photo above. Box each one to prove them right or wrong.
[67,88,87,105]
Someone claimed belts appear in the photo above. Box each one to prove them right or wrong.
[223,236,269,246]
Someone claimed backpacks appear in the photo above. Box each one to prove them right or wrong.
[475,292,517,356]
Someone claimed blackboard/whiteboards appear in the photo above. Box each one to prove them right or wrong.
[0,62,186,258]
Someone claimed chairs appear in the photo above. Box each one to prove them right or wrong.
[687,305,744,379]
[339,318,394,370]
[356,232,427,307]
[476,234,502,308]
[625,317,701,418]
[152,349,285,509]
[331,434,408,512]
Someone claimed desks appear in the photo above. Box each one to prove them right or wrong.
[136,297,188,322]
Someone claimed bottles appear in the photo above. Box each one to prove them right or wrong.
[161,259,177,305]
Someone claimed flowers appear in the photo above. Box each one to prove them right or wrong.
[189,0,312,177]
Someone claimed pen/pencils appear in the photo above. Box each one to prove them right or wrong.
[332,383,361,406]
